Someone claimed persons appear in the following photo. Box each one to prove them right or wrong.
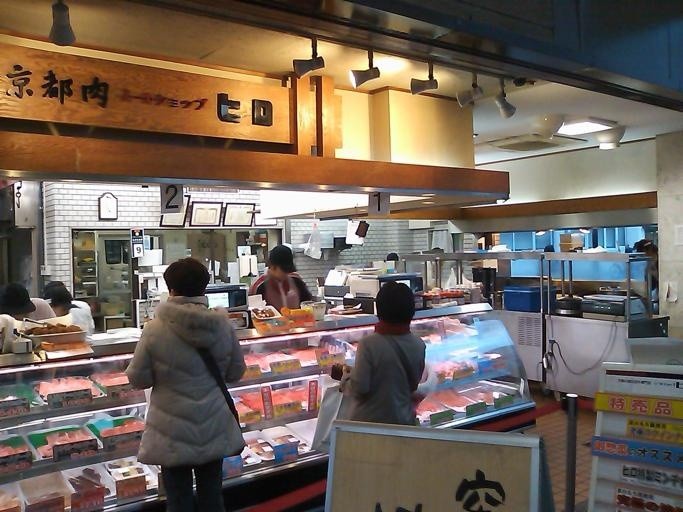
[537,245,561,277]
[248,244,314,316]
[426,247,444,289]
[123,256,248,512]
[0,279,95,356]
[339,279,428,427]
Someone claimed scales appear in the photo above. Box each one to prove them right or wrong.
[582,295,644,322]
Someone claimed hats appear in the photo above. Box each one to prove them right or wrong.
[269,245,296,272]
[43,282,73,306]
[1,283,36,316]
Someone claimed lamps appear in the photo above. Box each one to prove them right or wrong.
[292,38,519,120]
[532,109,627,152]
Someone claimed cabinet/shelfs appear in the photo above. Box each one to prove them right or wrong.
[1,304,538,512]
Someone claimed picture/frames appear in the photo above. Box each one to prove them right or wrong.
[97,192,257,228]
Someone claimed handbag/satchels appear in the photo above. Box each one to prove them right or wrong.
[311,385,343,452]
